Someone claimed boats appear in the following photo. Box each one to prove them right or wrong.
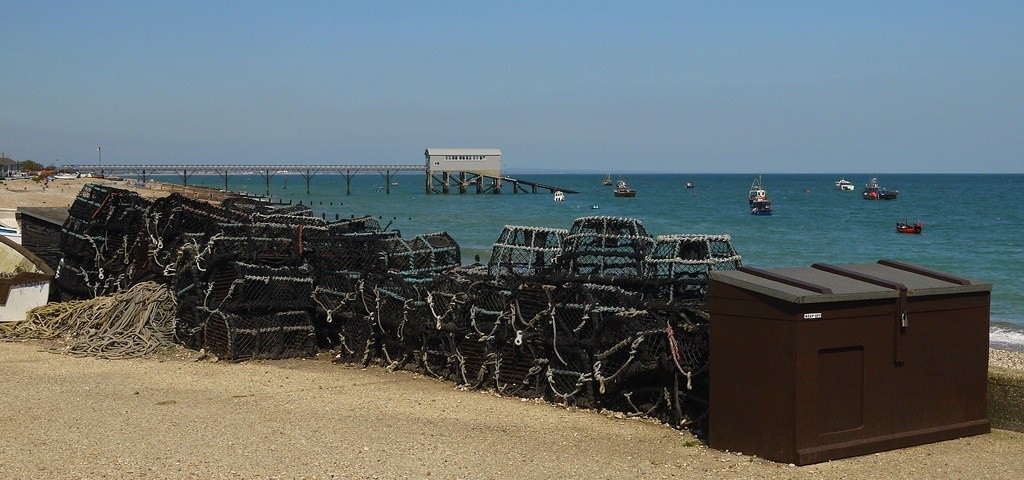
[590,203,600,209]
[896,216,924,234]
[613,172,637,198]
[747,173,774,216]
[554,190,565,202]
[685,180,694,188]
[603,172,611,183]
[11,170,124,181]
[862,177,900,202]
[834,178,855,192]
